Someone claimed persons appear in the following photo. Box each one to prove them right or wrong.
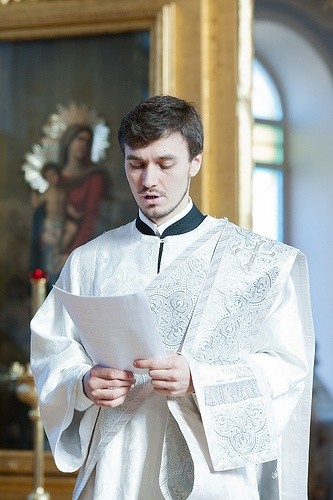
[29,95,306,499]
